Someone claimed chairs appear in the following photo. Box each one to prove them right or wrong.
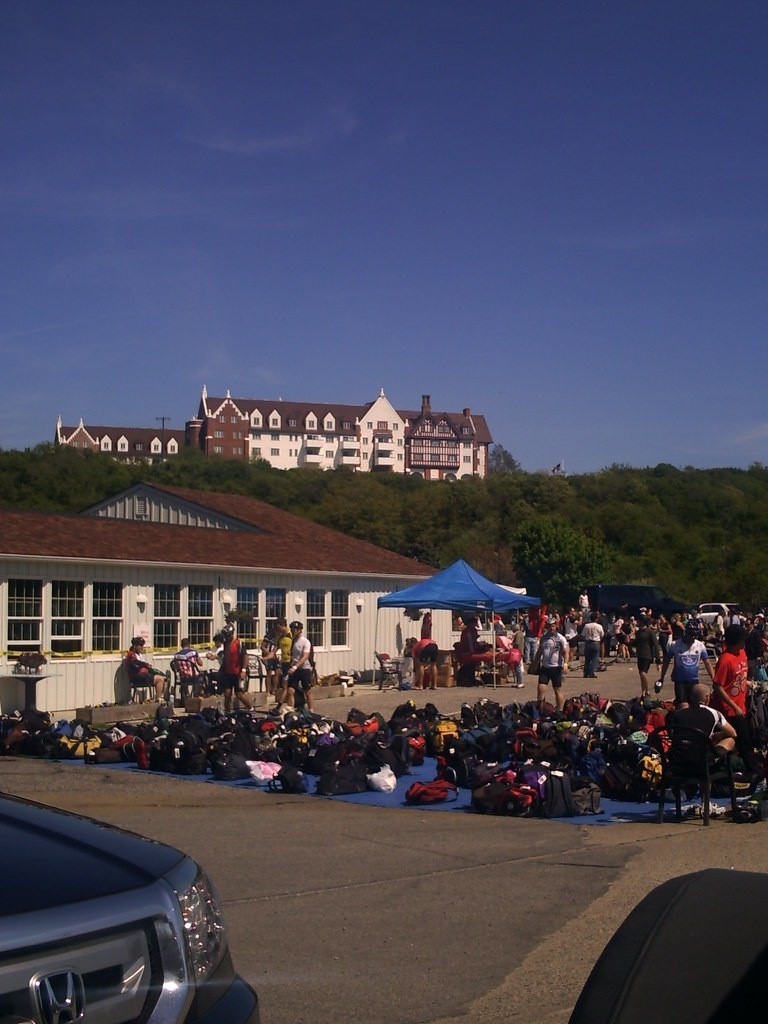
[246,654,266,693]
[652,724,738,826]
[170,658,207,698]
[122,659,156,703]
[373,650,402,691]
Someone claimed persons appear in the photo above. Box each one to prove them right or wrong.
[260,617,314,713]
[508,624,524,688]
[580,612,605,679]
[488,613,501,630]
[547,601,765,661]
[409,639,439,689]
[672,684,737,774]
[623,614,661,698]
[709,624,749,719]
[460,618,478,654]
[173,638,203,698]
[578,590,590,612]
[535,618,569,711]
[124,637,166,703]
[522,615,538,666]
[205,634,224,667]
[658,621,715,699]
[222,624,254,716]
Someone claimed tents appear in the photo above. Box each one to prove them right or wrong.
[373,560,541,689]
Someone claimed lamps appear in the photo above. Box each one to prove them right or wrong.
[222,595,233,603]
[295,597,304,606]
[355,599,364,606]
[136,593,149,603]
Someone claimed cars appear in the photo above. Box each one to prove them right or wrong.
[0,792,260,1024]
[687,603,744,625]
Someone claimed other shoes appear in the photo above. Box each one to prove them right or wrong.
[426,686,437,689]
[583,673,588,678]
[511,683,519,688]
[412,687,423,690]
[154,697,166,702]
[249,707,255,718]
[269,708,280,715]
[641,691,651,701]
[589,673,598,678]
[518,684,525,688]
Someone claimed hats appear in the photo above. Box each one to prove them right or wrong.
[755,613,765,619]
[690,610,697,615]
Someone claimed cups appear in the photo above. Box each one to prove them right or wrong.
[655,682,662,693]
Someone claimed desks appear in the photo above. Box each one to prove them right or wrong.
[462,652,517,688]
[0,674,64,710]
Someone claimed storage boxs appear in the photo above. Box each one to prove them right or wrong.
[439,665,455,677]
[426,676,458,688]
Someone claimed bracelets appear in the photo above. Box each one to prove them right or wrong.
[293,666,297,670]
[242,668,246,672]
[564,663,568,667]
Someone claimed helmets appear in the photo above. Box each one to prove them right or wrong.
[685,622,697,633]
[221,625,233,634]
[546,617,556,625]
[289,621,303,629]
[131,637,145,645]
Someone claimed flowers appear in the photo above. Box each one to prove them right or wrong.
[16,649,48,665]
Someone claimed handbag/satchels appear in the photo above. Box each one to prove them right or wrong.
[527,660,543,675]
[507,647,521,669]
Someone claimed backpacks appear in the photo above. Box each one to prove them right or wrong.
[0,688,768,826]
